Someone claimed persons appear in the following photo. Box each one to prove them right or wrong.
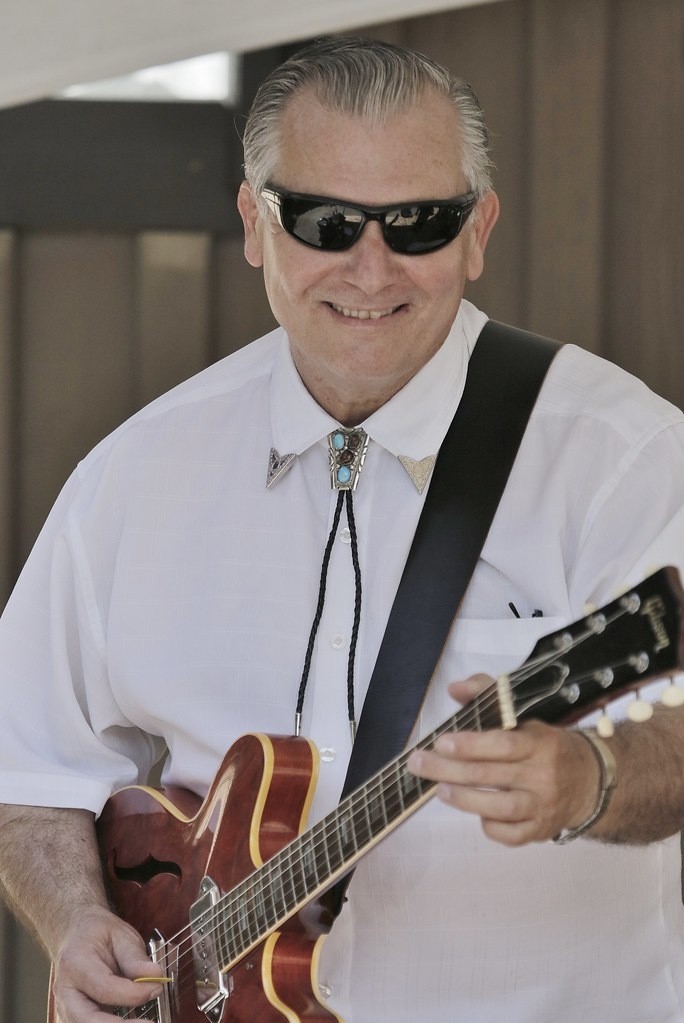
[0,38,684,1023]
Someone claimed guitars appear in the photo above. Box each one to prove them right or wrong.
[40,566,684,1023]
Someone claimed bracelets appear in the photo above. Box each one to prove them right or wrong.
[554,729,616,845]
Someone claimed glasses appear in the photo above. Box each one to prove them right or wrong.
[261,178,481,255]
